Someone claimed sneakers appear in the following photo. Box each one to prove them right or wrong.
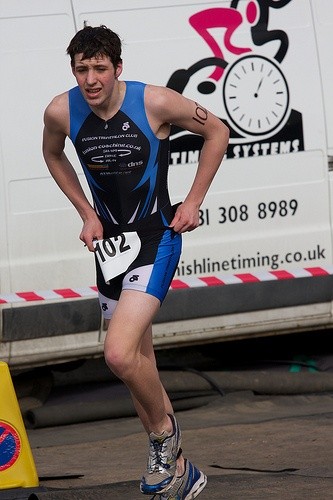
[148,458,208,500]
[139,414,182,495]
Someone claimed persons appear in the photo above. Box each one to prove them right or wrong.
[42,26,231,500]
